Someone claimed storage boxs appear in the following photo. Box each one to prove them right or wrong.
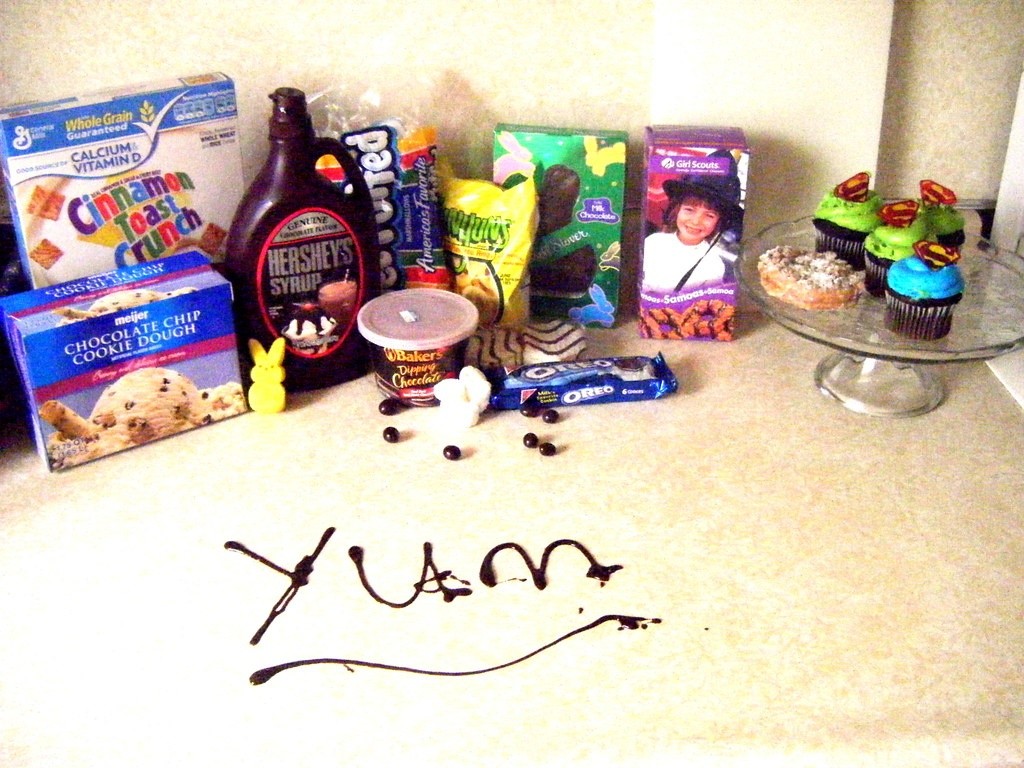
[0,250,249,475]
[0,71,246,289]
[493,124,629,329]
[635,123,750,342]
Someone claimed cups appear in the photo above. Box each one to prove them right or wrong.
[356,284,478,409]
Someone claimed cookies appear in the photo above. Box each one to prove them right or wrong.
[614,359,644,371]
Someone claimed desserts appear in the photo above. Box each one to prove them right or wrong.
[49,286,197,328]
[33,367,246,471]
[812,172,966,341]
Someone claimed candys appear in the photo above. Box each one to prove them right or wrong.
[379,399,559,460]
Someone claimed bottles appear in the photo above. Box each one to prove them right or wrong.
[227,89,380,391]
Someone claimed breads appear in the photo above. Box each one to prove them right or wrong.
[757,245,865,312]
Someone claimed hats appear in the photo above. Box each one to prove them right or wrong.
[662,149,743,231]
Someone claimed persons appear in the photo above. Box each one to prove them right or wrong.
[643,149,744,292]
